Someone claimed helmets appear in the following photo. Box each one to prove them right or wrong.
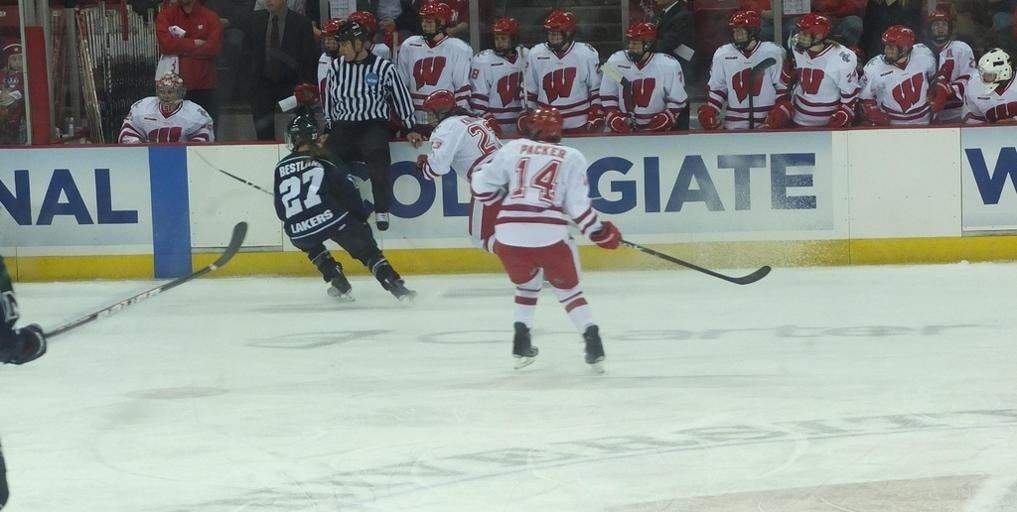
[156,74,186,103]
[322,11,379,54]
[423,89,457,113]
[527,106,563,141]
[925,9,954,34]
[418,3,454,27]
[544,11,576,33]
[287,115,318,151]
[490,15,521,35]
[882,24,916,50]
[728,10,763,32]
[626,19,660,48]
[3,44,23,71]
[795,13,831,37]
[978,47,1013,94]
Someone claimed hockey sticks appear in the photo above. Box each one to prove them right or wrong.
[43,222,246,340]
[747,57,777,128]
[598,62,638,129]
[619,239,770,285]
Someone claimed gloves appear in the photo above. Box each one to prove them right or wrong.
[591,219,622,250]
[648,109,676,132]
[697,104,722,130]
[517,109,530,134]
[764,99,794,128]
[828,99,890,128]
[926,78,953,111]
[8,324,47,365]
[587,104,632,133]
[482,112,502,138]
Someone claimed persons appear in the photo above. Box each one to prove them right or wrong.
[321,20,427,232]
[271,113,418,304]
[0,253,50,512]
[469,107,623,366]
[417,87,554,287]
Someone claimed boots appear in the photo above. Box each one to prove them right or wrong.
[376,210,390,231]
[324,262,353,297]
[377,266,417,300]
[583,322,606,364]
[513,321,541,358]
